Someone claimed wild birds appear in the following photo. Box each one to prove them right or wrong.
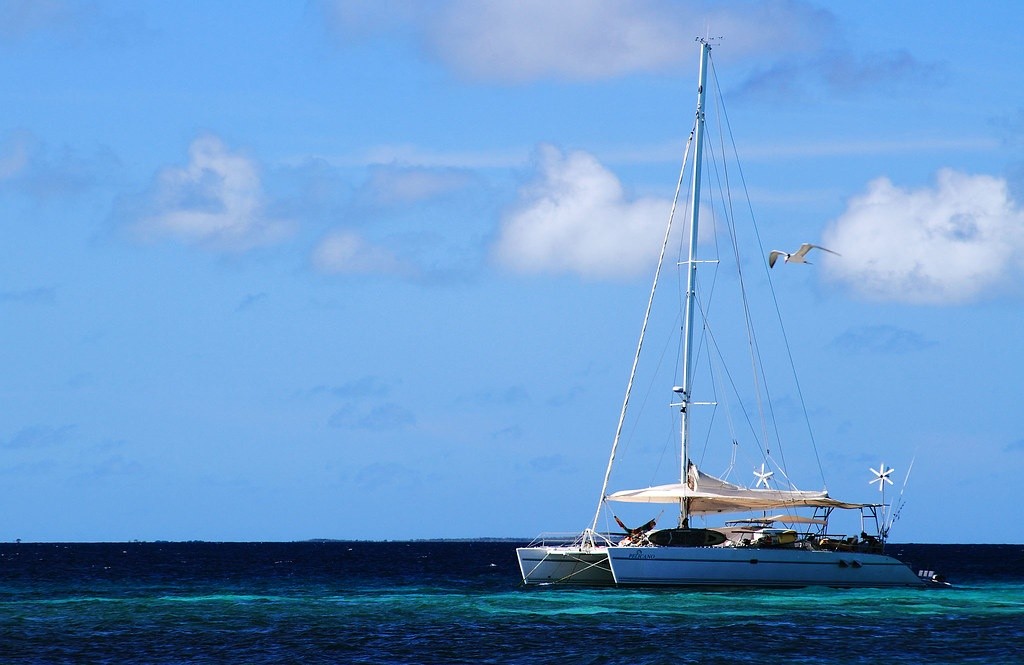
[769,242,842,269]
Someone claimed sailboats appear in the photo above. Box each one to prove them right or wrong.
[517,24,863,591]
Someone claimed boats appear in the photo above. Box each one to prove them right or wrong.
[603,462,950,590]
